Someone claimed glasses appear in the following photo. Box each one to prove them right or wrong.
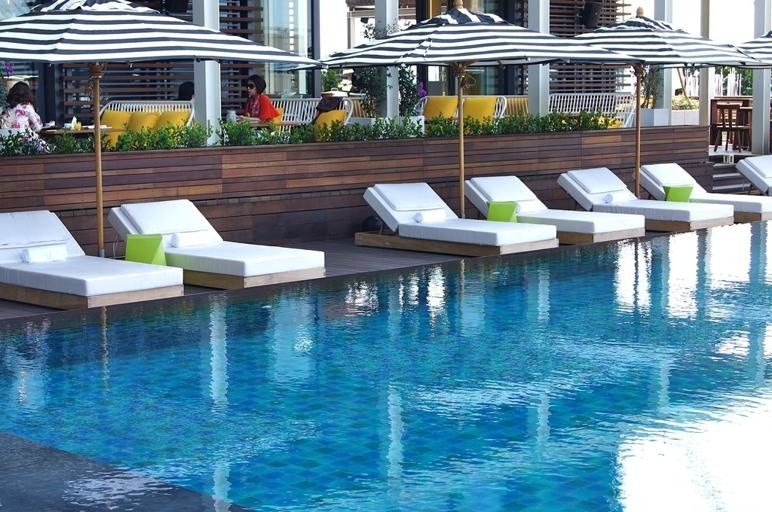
[246,84,254,89]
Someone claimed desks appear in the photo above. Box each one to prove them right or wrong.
[38,126,122,136]
[122,234,167,268]
[486,198,521,225]
[661,183,694,203]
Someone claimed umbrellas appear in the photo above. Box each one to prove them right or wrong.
[568,7,765,200]
[733,28,772,67]
[314,0,645,219]
[0,0,328,258]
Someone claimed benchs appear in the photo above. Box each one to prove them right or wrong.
[98,99,194,147]
[269,92,636,142]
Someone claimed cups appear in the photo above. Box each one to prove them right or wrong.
[74,122,81,131]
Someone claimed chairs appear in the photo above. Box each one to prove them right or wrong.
[354,179,558,258]
[553,165,737,231]
[0,208,185,308]
[461,175,646,245]
[713,103,750,154]
[733,155,772,197]
[635,162,772,222]
[107,196,326,287]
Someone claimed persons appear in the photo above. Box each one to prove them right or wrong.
[238,74,279,132]
[174,82,196,109]
[0,81,57,155]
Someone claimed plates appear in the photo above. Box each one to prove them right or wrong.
[87,125,112,130]
[238,118,261,123]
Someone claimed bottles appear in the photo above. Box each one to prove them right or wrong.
[226,109,236,124]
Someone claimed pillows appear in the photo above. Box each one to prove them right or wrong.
[101,109,190,147]
